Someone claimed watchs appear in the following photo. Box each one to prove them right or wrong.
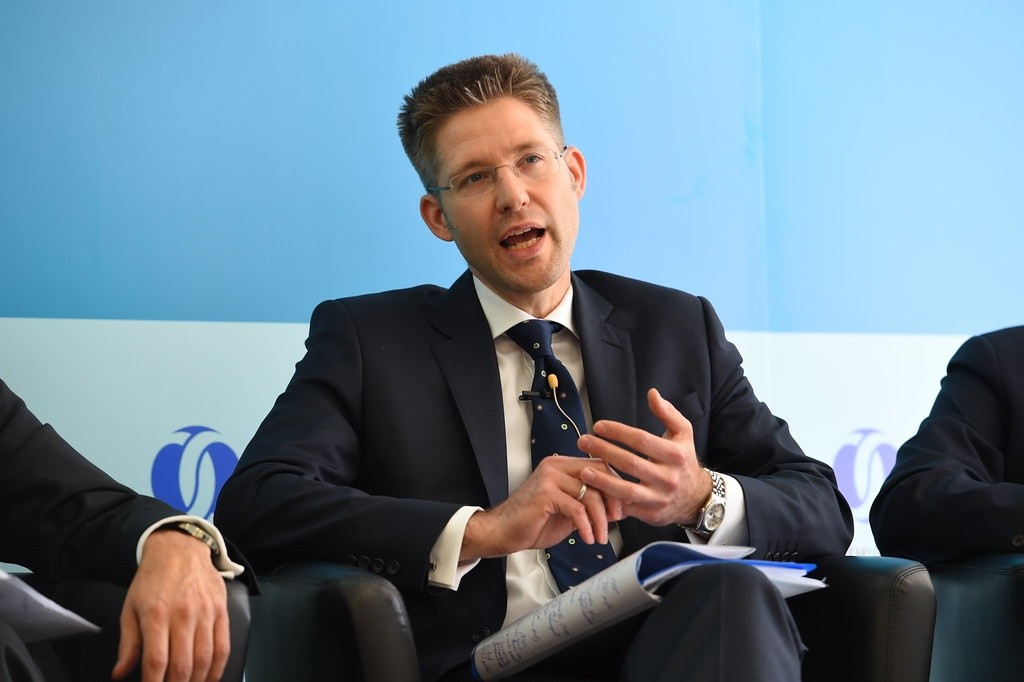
[161,522,219,554]
[677,467,726,538]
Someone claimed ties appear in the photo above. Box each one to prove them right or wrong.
[505,319,618,594]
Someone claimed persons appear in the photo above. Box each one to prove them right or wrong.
[0,377,260,682]
[214,53,855,682]
[868,324,1024,560]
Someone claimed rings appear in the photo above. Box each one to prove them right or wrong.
[576,482,587,501]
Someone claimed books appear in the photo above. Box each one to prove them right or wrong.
[471,541,830,682]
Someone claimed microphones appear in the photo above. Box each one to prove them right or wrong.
[548,374,559,389]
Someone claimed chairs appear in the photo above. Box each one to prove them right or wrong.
[924,547,1024,682]
[6,572,254,681]
[244,557,938,682]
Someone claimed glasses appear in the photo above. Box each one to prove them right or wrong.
[427,146,567,200]
